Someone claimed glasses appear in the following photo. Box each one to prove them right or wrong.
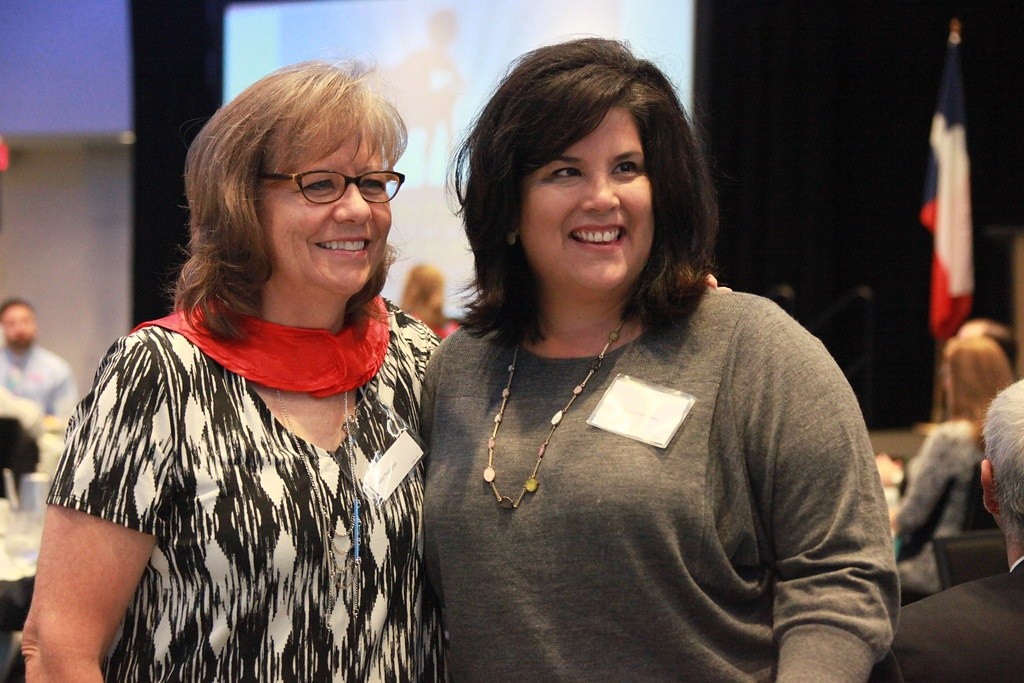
[256,169,405,203]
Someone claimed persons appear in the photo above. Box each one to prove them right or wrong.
[22,63,730,682]
[401,265,458,339]
[869,381,1023,683]
[420,39,899,683]
[890,321,1017,602]
[0,297,75,498]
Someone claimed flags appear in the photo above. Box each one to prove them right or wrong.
[922,52,973,337]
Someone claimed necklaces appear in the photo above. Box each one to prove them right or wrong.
[484,322,627,509]
[277,390,359,640]
